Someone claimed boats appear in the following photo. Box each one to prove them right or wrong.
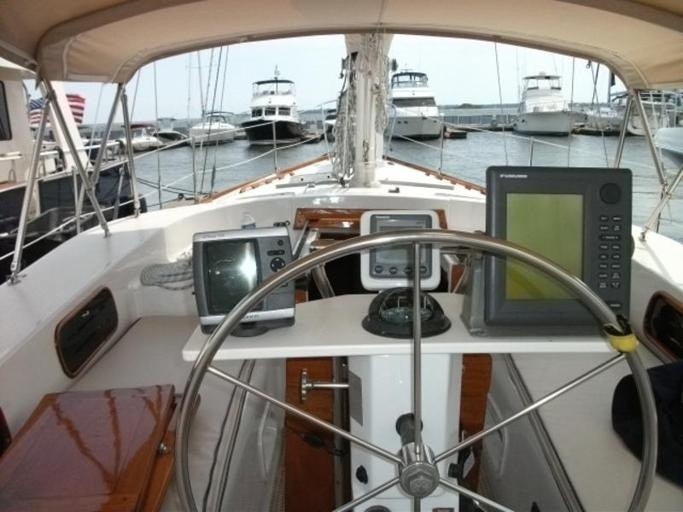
[242,69,307,147]
[515,74,574,137]
[384,67,443,142]
[585,86,683,139]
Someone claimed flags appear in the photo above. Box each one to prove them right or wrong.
[27,92,87,129]
